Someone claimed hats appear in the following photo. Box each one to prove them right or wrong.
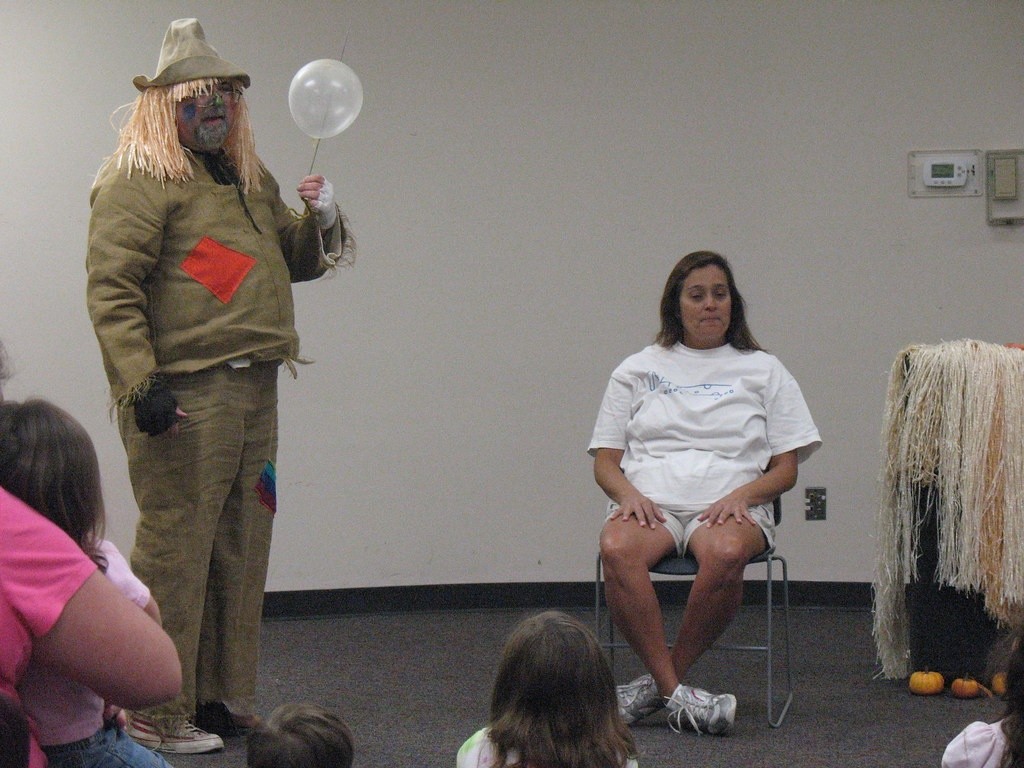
[133,18,250,93]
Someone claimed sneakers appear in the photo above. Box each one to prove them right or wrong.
[662,682,736,735]
[615,672,662,728]
[125,712,224,754]
[193,698,260,733]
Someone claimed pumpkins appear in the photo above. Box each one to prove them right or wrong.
[909,665,945,695]
[991,671,1008,695]
[951,671,979,699]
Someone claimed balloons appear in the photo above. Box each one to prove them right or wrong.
[288,59,363,139]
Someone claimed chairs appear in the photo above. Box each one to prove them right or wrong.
[594,454,795,728]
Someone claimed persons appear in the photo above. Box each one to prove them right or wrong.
[457,610,637,768]
[586,252,822,734]
[246,705,352,767]
[85,18,350,754]
[0,398,182,768]
[941,635,1024,768]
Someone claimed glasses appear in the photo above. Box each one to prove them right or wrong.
[180,90,242,109]
